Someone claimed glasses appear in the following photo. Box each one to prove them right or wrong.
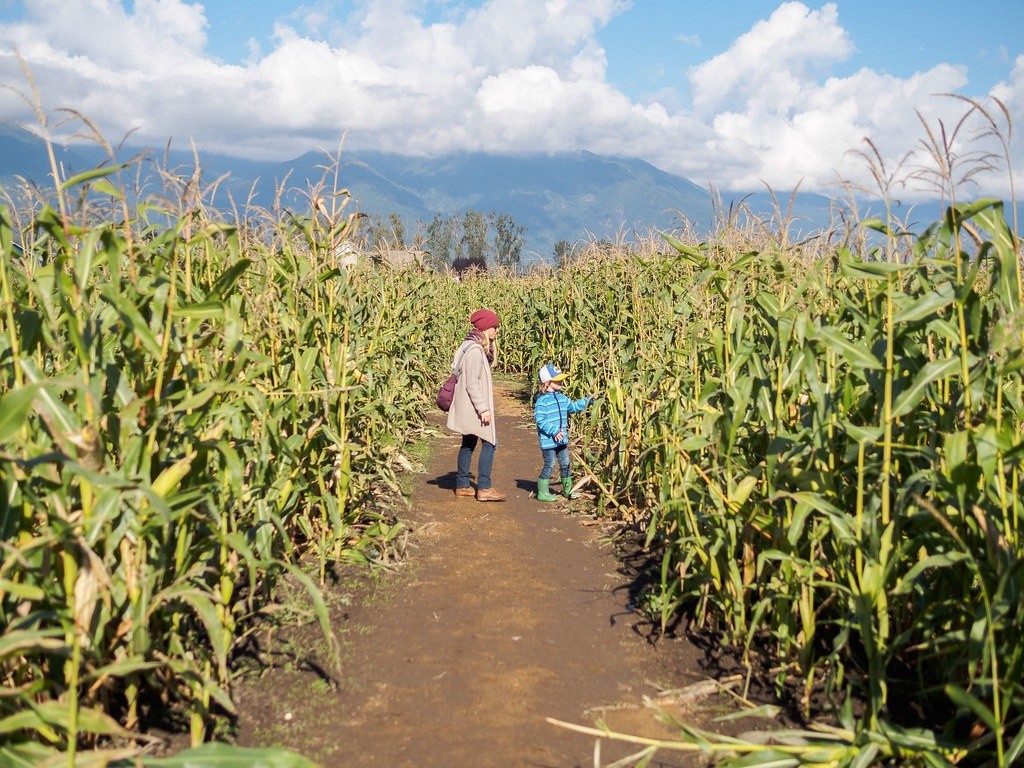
[492,325,499,330]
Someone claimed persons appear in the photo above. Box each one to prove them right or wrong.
[447,309,508,501]
[532,364,598,502]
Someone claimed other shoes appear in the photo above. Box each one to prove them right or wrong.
[455,484,477,496]
[477,487,507,501]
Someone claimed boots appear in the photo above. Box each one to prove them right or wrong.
[561,477,580,499]
[536,477,559,502]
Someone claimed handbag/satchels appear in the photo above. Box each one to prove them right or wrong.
[436,373,459,414]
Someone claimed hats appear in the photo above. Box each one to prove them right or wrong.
[470,309,500,334]
[538,365,569,383]
